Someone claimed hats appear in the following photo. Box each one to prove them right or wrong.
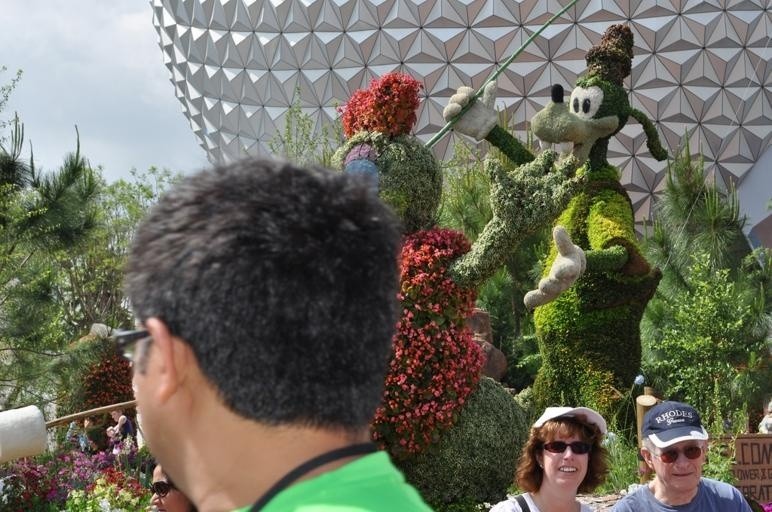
[534,406,607,437]
[642,402,709,448]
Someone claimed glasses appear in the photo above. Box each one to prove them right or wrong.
[658,446,701,464]
[542,440,592,455]
[116,330,152,361]
[149,482,173,498]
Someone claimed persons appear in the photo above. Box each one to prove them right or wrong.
[82,424,109,455]
[106,154,441,512]
[103,404,135,445]
[598,397,756,512]
[65,418,82,442]
[150,458,200,511]
[77,414,98,454]
[481,401,618,511]
